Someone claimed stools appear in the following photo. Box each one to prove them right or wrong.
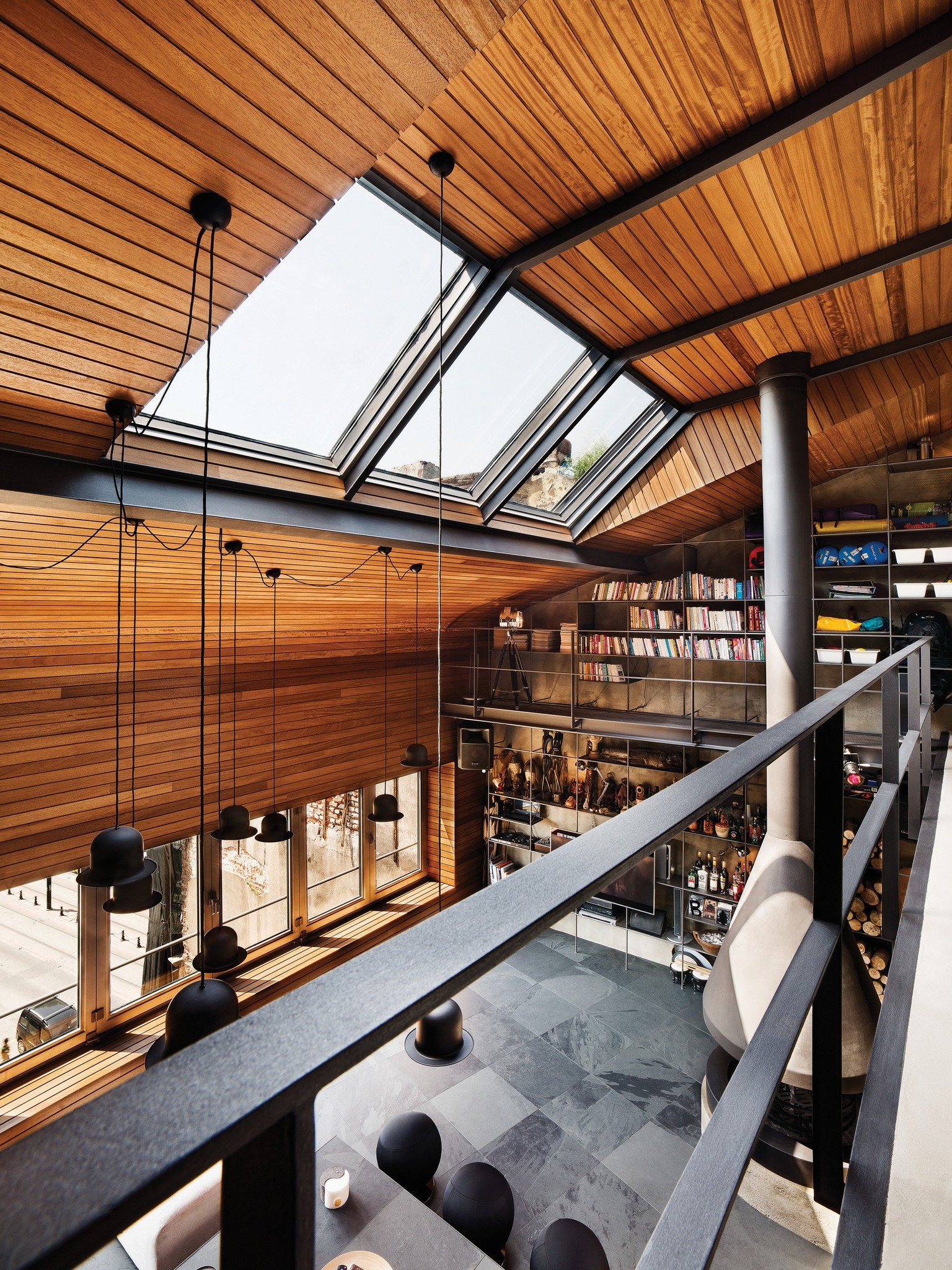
[376,1110,442,1209]
[530,1217,611,1270]
[442,1161,514,1268]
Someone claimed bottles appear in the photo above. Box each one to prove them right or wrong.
[688,801,767,846]
[688,850,752,902]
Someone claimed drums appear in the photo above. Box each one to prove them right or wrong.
[670,962,691,986]
[691,969,711,991]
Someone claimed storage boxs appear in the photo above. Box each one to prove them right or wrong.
[892,548,928,564]
[893,583,931,598]
[929,583,952,598]
[692,929,727,956]
[928,547,952,563]
[814,647,847,663]
[847,649,881,664]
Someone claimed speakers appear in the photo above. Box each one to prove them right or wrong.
[666,888,693,944]
[630,908,667,937]
[656,839,676,880]
[457,721,494,770]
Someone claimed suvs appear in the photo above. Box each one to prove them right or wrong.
[15,996,78,1054]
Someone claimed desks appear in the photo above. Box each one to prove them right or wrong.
[165,1135,510,1270]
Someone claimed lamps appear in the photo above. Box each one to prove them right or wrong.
[68,149,523,1073]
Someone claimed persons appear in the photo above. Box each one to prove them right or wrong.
[495,731,627,818]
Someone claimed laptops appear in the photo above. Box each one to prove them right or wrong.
[503,799,541,825]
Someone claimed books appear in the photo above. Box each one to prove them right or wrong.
[485,570,766,684]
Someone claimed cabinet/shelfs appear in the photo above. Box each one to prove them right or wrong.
[475,722,889,1013]
[483,453,952,724]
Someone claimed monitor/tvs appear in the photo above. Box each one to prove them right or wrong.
[550,826,655,915]
